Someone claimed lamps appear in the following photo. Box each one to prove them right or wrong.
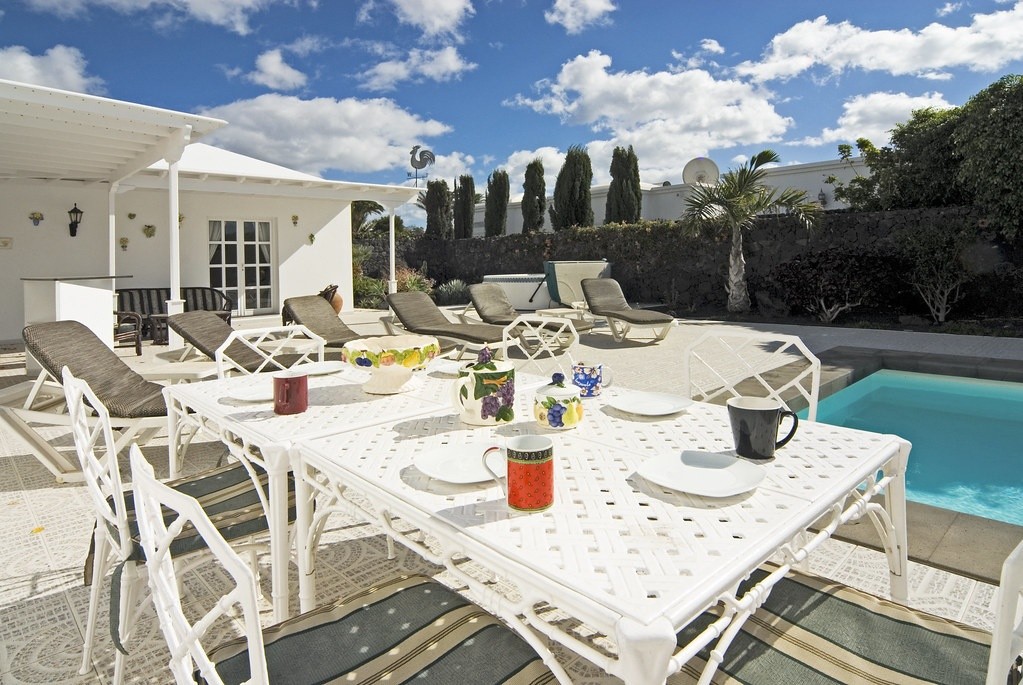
[67,202,84,237]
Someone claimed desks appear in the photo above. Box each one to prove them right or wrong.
[288,381,912,685]
[667,540,1023,685]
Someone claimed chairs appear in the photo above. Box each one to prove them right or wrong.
[126,444,570,685]
[282,284,456,359]
[378,291,524,362]
[683,329,821,424]
[59,366,319,685]
[0,320,232,484]
[463,278,678,381]
[165,309,345,383]
[113,310,143,356]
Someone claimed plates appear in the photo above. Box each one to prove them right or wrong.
[294,361,345,374]
[609,391,692,416]
[227,374,273,401]
[414,441,505,483]
[636,452,760,498]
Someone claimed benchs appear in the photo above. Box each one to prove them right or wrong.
[115,287,234,338]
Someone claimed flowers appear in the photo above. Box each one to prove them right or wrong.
[292,214,298,222]
[143,225,156,238]
[28,212,44,221]
[120,237,130,245]
[309,233,316,242]
[178,213,186,222]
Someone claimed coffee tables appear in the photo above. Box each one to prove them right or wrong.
[148,311,232,345]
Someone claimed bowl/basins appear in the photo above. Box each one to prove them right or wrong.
[341,336,440,395]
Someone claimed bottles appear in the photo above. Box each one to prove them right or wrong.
[534,372,582,430]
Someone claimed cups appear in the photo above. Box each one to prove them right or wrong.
[273,371,309,416]
[726,396,798,459]
[482,435,554,511]
[572,364,613,399]
[456,348,516,425]
